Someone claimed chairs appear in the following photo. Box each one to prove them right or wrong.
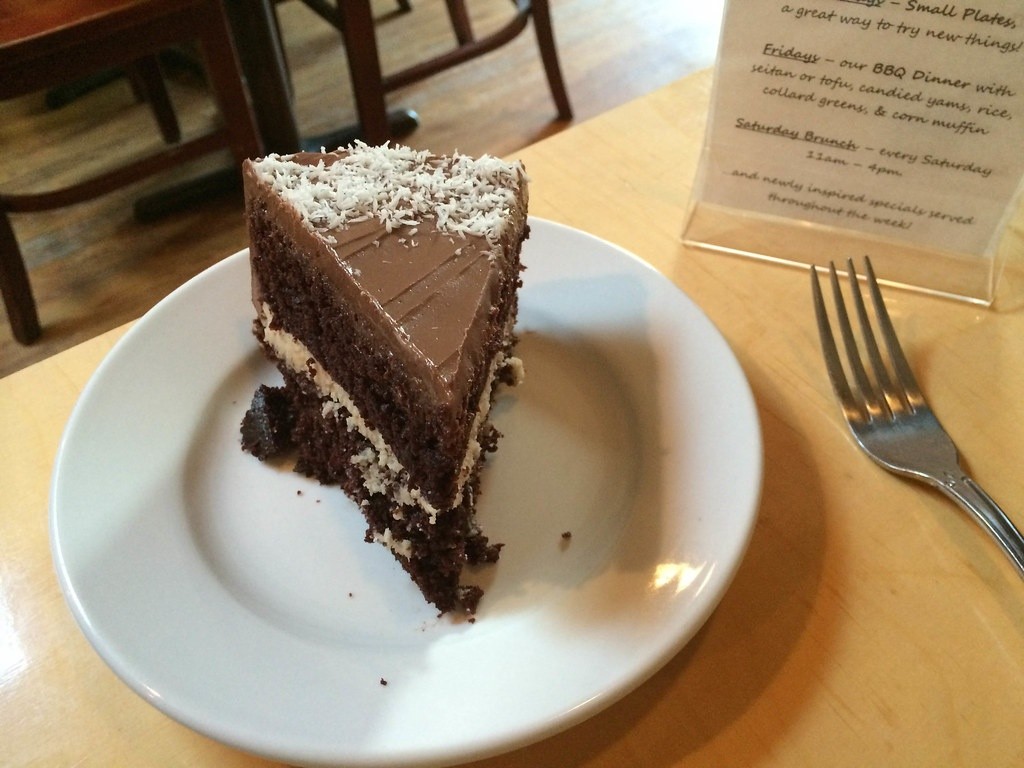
[0,0,574,348]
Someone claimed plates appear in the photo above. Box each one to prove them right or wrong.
[51,216,764,768]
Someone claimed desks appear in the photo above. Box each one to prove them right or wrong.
[0,61,1024,768]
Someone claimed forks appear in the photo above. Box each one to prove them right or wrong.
[808,256,1024,580]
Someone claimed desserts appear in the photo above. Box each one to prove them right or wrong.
[228,139,532,616]
